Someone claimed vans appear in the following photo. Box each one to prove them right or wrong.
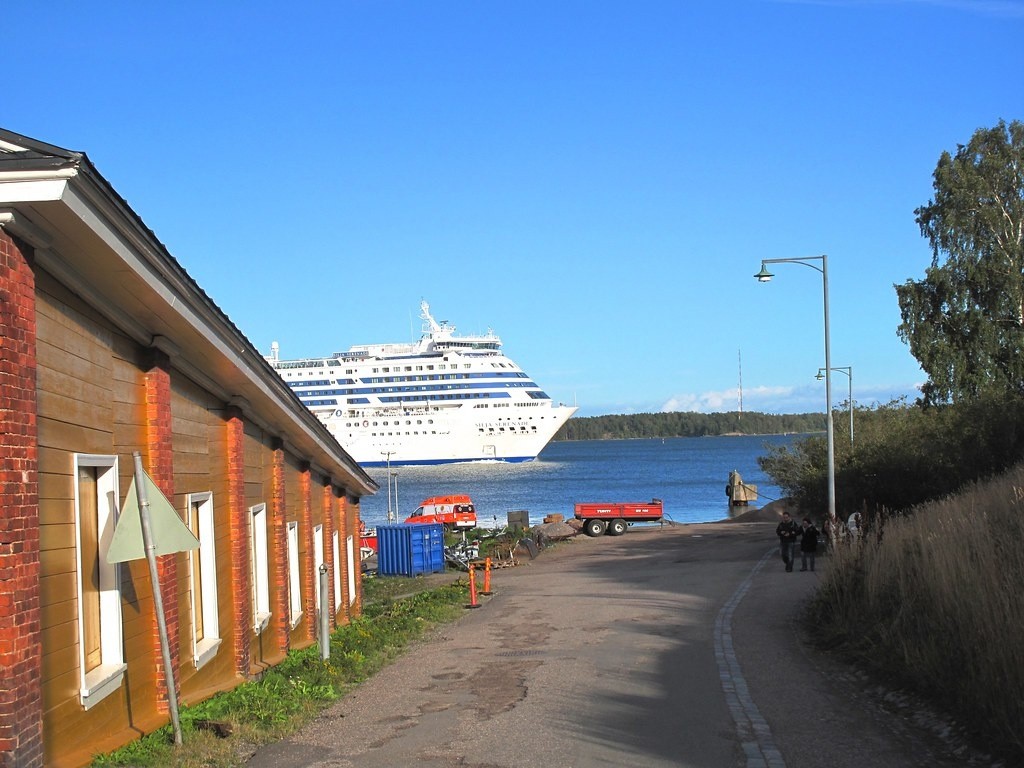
[402,494,476,527]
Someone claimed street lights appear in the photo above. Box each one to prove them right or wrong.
[753,253,837,518]
[384,450,396,523]
[390,472,399,526]
[814,366,856,467]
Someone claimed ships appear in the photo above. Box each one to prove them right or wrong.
[262,296,580,468]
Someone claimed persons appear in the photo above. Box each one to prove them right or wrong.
[360,521,365,532]
[823,511,885,556]
[776,512,820,572]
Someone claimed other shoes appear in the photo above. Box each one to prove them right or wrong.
[785,561,793,572]
[811,568,815,571]
[800,568,807,571]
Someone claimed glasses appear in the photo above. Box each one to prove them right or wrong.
[784,517,788,519]
